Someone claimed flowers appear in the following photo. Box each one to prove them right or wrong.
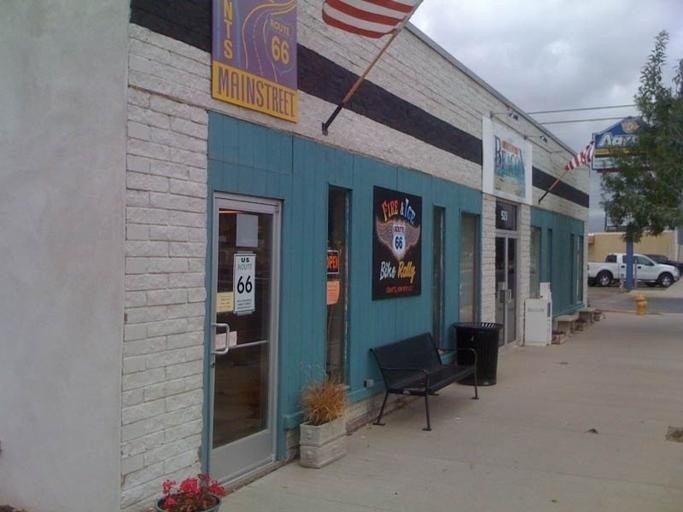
[163,474,227,511]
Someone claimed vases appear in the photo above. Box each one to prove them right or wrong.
[156,494,222,512]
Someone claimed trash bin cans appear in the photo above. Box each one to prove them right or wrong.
[453,321,504,386]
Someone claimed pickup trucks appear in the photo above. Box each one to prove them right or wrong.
[587,252,680,288]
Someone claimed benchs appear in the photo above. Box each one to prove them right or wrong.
[369,332,479,431]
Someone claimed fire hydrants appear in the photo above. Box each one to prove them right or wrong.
[635,294,648,316]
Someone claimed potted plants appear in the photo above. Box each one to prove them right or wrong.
[299,364,347,469]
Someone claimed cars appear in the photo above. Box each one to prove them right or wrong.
[501,260,513,271]
[640,254,682,280]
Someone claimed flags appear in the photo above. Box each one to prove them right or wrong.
[565,141,595,170]
[321,0,422,39]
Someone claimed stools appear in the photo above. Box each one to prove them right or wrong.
[578,307,595,323]
[555,316,578,336]
